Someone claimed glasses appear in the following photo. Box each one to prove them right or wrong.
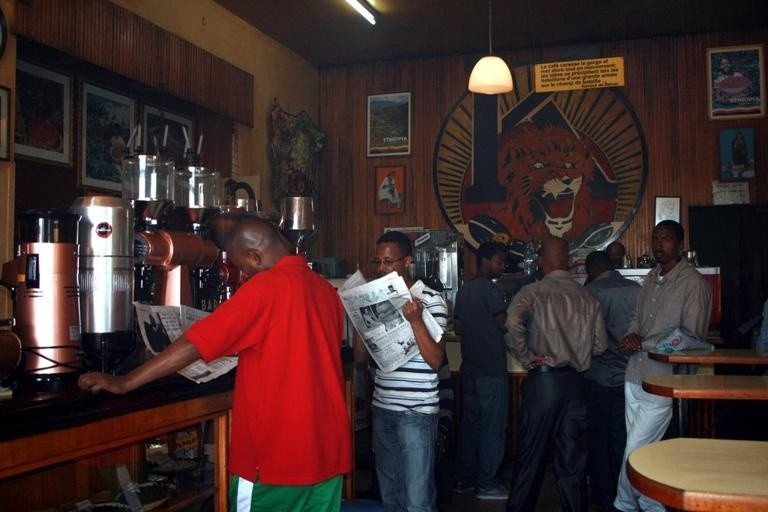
[372,258,404,266]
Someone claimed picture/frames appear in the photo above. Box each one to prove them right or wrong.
[0,87,10,162]
[653,196,681,227]
[77,77,139,196]
[367,92,412,157]
[14,58,73,167]
[718,127,757,181]
[705,43,766,122]
[375,165,406,215]
[711,179,751,206]
[141,99,196,167]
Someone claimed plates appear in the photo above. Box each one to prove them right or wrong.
[80,479,176,512]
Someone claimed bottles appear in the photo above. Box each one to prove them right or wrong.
[623,254,632,268]
[523,235,543,274]
[732,131,747,165]
[422,255,444,292]
[687,249,699,267]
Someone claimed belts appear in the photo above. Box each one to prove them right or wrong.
[528,365,571,376]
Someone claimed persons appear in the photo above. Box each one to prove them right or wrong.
[353,231,448,512]
[452,243,511,499]
[714,58,751,107]
[722,132,755,180]
[505,221,714,512]
[79,222,351,512]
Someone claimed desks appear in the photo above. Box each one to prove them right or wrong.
[626,348,768,512]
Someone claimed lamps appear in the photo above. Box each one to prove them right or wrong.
[345,0,377,26]
[468,0,514,94]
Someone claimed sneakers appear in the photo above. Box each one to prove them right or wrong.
[454,481,509,500]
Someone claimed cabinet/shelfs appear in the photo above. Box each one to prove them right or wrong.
[0,343,355,512]
